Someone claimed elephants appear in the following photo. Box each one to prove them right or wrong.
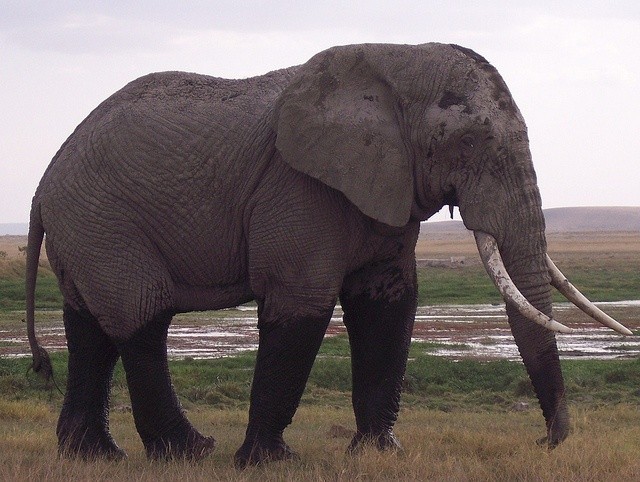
[24,42,634,472]
